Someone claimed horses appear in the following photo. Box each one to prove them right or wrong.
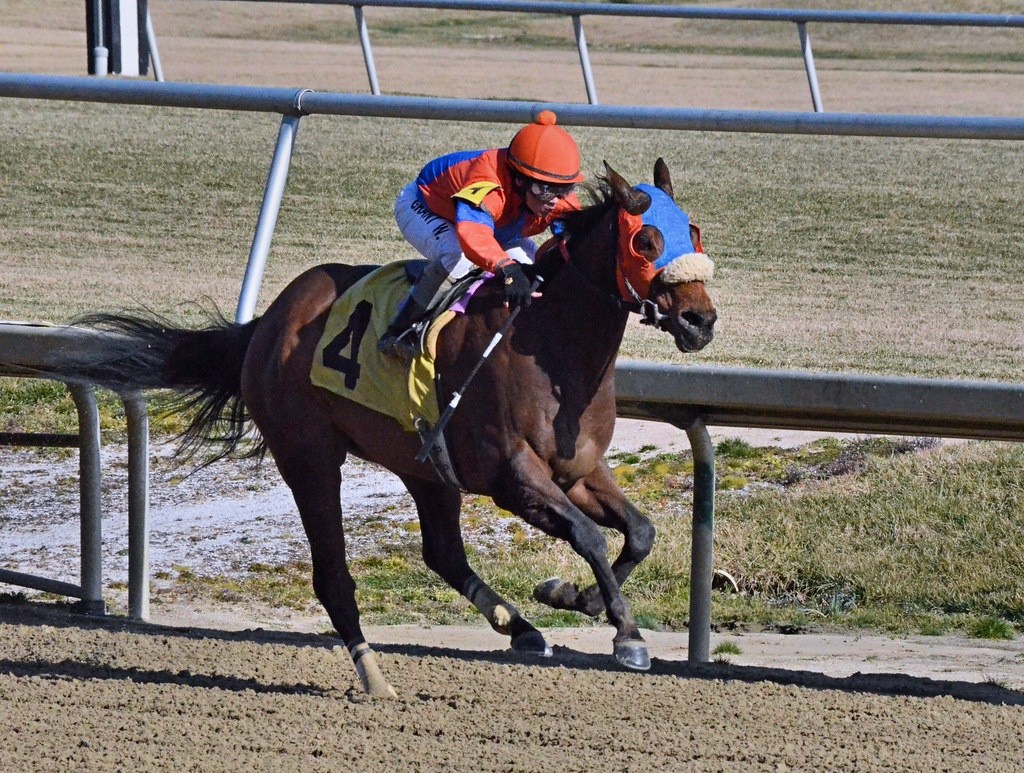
[47,150,723,710]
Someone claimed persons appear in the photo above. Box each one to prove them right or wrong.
[377,111,585,360]
[86,0,149,75]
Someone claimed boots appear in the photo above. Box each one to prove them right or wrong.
[378,296,426,358]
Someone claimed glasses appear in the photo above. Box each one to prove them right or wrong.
[527,180,576,203]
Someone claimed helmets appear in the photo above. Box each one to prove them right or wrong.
[507,112,584,184]
